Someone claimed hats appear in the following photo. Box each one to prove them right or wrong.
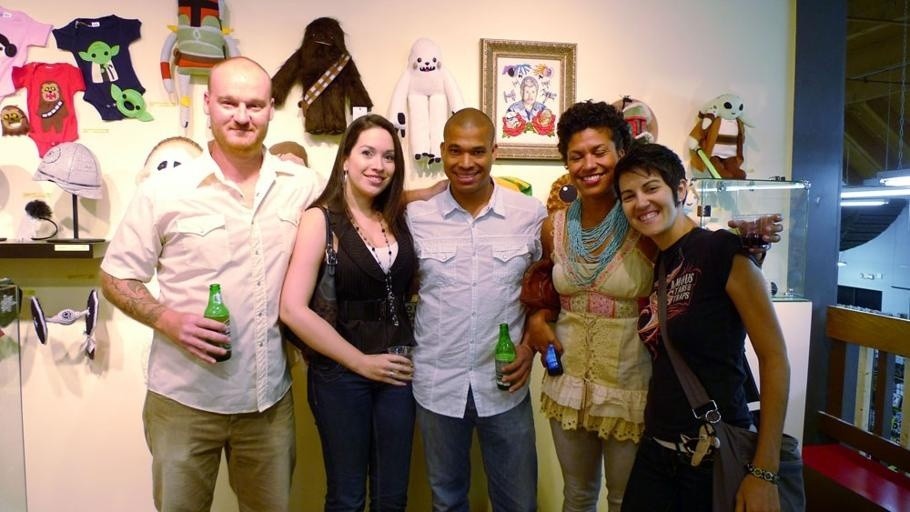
[31,140,106,203]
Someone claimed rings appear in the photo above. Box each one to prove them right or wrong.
[390,370,395,378]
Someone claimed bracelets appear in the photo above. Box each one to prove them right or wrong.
[744,463,781,485]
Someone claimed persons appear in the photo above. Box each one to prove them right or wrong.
[507,77,546,121]
[613,143,791,511]
[406,108,549,512]
[100,58,448,511]
[278,115,420,512]
[527,98,783,511]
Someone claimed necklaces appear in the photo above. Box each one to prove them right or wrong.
[567,197,631,288]
[349,208,401,328]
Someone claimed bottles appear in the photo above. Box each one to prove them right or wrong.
[546,343,563,377]
[200,283,234,363]
[493,321,519,393]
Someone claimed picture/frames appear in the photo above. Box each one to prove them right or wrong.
[479,37,577,161]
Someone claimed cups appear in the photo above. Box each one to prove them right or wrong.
[732,212,774,251]
[385,345,417,380]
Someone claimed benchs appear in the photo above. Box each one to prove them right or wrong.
[803,303,910,512]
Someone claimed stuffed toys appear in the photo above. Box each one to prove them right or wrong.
[161,1,241,131]
[689,93,758,179]
[267,17,374,136]
[389,40,462,167]
[623,98,655,143]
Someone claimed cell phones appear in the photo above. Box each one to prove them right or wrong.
[544,344,563,376]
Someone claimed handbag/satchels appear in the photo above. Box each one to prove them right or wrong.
[280,248,342,357]
[707,415,809,511]
[517,257,562,311]
